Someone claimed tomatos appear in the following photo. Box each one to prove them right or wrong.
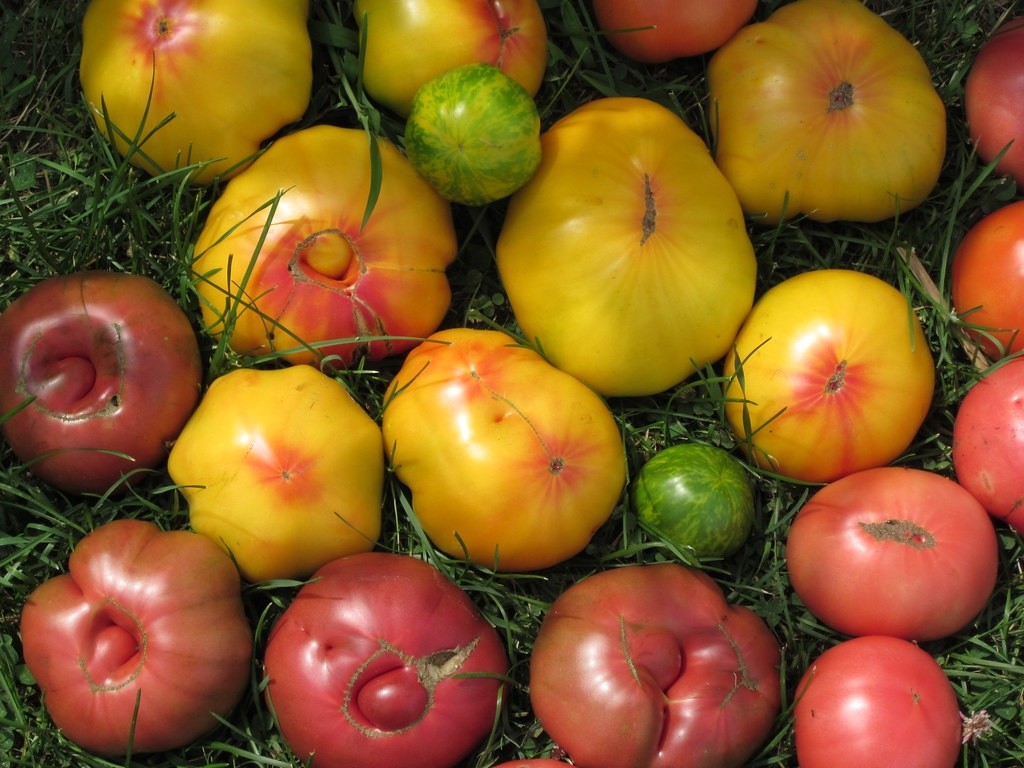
[0,0,1024,768]
